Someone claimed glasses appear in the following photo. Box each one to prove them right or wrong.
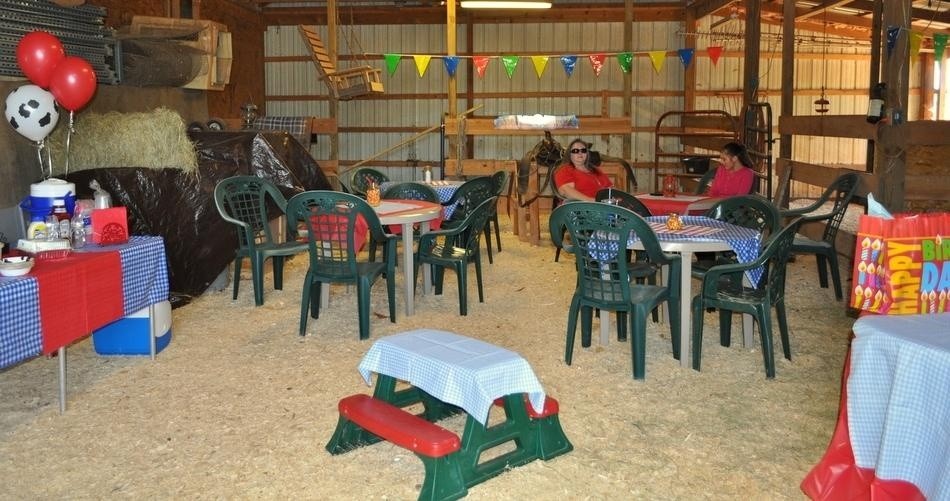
[567,146,588,154]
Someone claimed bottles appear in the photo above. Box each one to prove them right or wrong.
[241,104,260,130]
[866,81,885,123]
[424,166,431,185]
[51,200,71,241]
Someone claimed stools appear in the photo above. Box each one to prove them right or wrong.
[494,397,575,464]
[323,393,470,501]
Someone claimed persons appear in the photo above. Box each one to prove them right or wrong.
[552,138,615,204]
[700,142,754,197]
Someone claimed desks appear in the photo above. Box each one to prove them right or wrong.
[800,312,950,501]
[358,329,541,489]
[0,235,171,415]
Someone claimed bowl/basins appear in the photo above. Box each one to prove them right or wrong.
[0,256,35,276]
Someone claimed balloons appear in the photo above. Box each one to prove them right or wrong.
[5,85,59,141]
[51,56,97,111]
[16,31,65,88]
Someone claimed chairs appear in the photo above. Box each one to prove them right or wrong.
[696,165,763,195]
[440,175,493,263]
[691,216,806,379]
[380,183,439,286]
[548,200,682,379]
[286,190,397,339]
[551,164,569,263]
[350,166,391,196]
[214,174,309,307]
[691,194,780,281]
[484,169,509,264]
[414,195,499,316]
[780,172,865,302]
[595,187,661,341]
